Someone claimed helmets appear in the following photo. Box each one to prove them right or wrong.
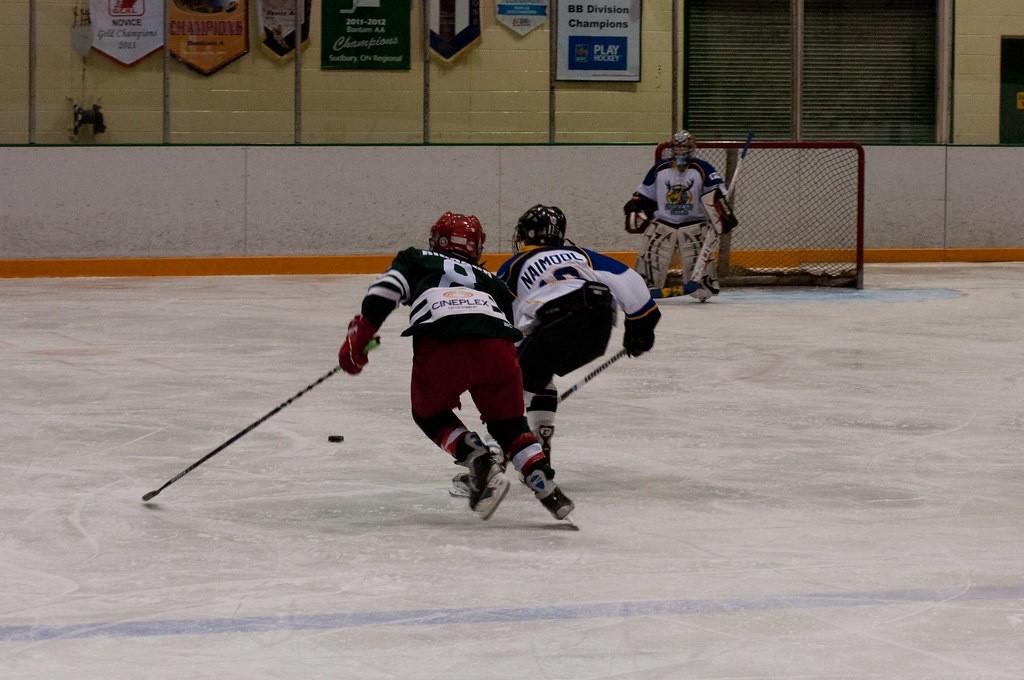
[429,210,486,262]
[512,204,566,255]
[672,129,696,165]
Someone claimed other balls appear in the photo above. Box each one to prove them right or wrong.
[327,435,345,443]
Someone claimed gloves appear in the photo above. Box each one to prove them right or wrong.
[623,319,654,358]
[339,315,375,375]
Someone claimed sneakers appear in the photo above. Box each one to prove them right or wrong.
[523,462,579,530]
[449,447,508,499]
[455,430,510,519]
[531,423,555,465]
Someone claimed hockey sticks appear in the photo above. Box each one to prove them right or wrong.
[649,134,754,301]
[555,340,630,405]
[141,334,383,502]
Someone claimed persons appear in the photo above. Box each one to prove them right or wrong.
[337,210,578,529]
[449,204,662,497]
[622,129,739,303]
